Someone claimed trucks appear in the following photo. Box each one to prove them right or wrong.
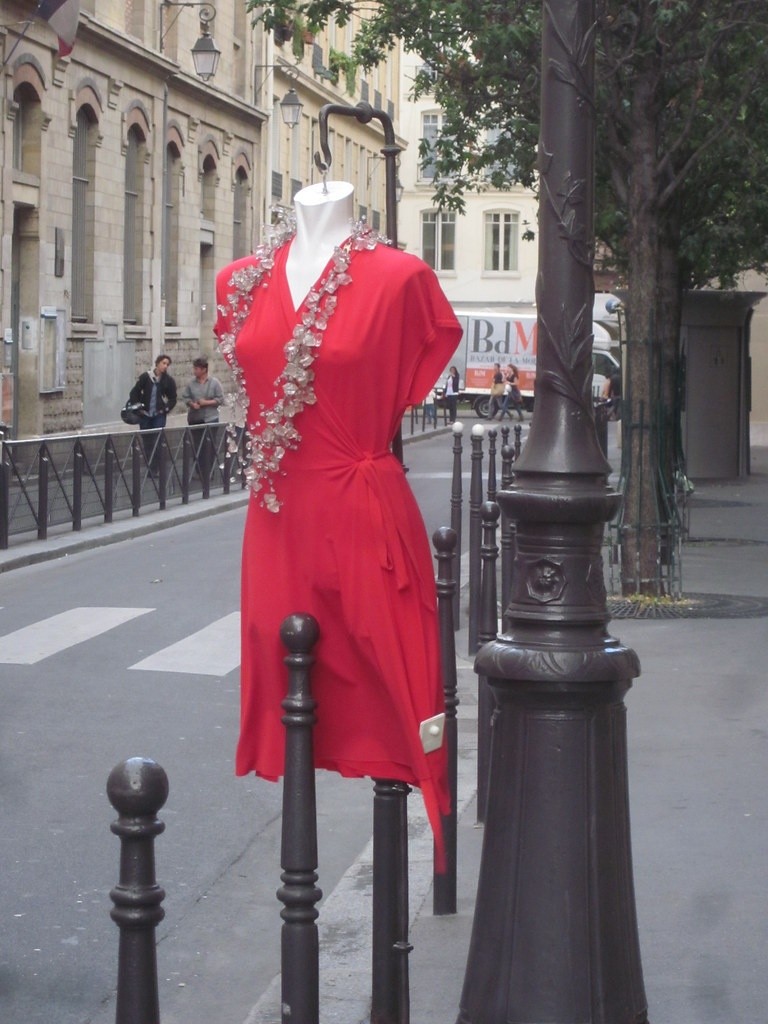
[424,311,621,417]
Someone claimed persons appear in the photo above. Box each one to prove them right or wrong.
[444,366,459,424]
[421,388,437,424]
[129,355,177,478]
[602,371,622,421]
[182,358,224,480]
[498,364,524,422]
[487,363,514,421]
[216,179,464,875]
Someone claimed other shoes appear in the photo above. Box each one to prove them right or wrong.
[447,421,454,426]
[146,466,159,479]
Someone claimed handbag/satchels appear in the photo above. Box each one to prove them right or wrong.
[512,395,524,406]
[121,400,145,426]
[490,382,504,395]
